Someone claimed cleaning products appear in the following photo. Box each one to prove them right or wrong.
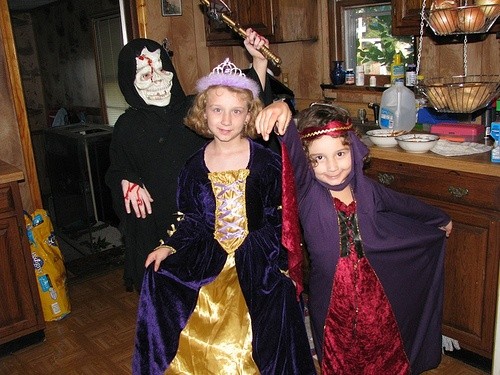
[391,54,405,86]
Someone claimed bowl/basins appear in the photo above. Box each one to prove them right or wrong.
[395,133,441,154]
[366,128,409,148]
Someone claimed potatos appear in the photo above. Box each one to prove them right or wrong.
[428,83,490,111]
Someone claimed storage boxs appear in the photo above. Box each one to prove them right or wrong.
[429,122,485,143]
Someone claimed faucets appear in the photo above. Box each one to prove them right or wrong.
[360,103,381,124]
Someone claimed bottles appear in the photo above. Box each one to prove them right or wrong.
[329,60,376,86]
[389,54,417,87]
[378,79,417,133]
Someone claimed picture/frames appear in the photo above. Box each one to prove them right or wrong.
[161,0,183,17]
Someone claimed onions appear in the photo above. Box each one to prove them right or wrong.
[429,0,500,32]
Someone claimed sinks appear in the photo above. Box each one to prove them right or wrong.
[353,123,381,140]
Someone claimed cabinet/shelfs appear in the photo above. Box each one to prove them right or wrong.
[204,0,318,46]
[363,156,500,359]
[0,181,46,347]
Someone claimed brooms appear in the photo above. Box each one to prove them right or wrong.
[80,131,108,230]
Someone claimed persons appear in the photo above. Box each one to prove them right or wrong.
[133,59,317,375]
[106,28,295,292]
[255,102,452,375]
